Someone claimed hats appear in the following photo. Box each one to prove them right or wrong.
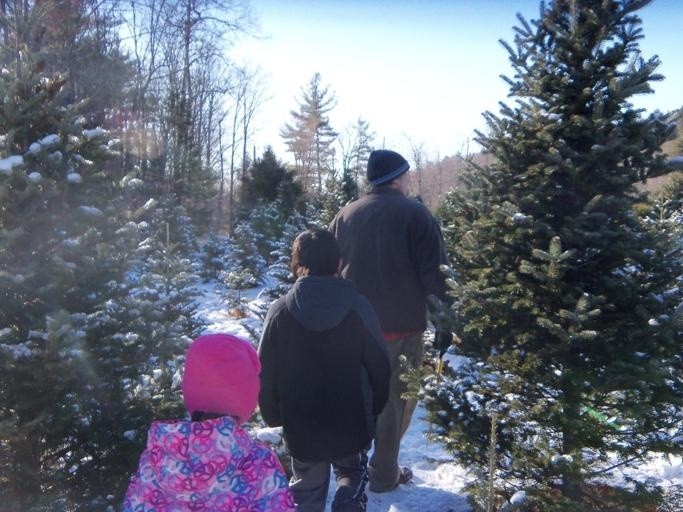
[182,333,262,424]
[368,151,409,186]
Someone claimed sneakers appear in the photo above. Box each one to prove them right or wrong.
[335,501,362,512]
[397,467,412,484]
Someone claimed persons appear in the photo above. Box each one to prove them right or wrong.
[121,334,297,512]
[255,229,391,512]
[328,149,456,494]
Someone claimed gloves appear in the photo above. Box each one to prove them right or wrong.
[433,332,453,358]
